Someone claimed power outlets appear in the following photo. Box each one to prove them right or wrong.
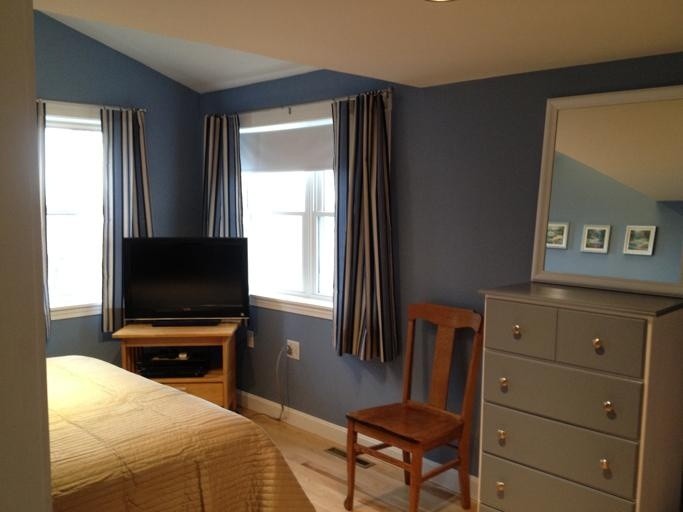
[285,338,300,360]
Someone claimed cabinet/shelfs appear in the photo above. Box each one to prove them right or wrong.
[475,281,683,512]
[111,323,240,411]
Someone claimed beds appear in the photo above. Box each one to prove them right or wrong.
[44,353,282,512]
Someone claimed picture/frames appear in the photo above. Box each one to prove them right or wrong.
[580,223,612,254]
[544,220,570,249]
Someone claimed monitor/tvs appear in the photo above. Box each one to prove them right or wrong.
[121,237,250,327]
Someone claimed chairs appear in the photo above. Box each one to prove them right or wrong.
[343,301,485,510]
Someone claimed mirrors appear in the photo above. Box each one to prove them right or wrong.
[529,85,682,299]
[622,225,657,256]
